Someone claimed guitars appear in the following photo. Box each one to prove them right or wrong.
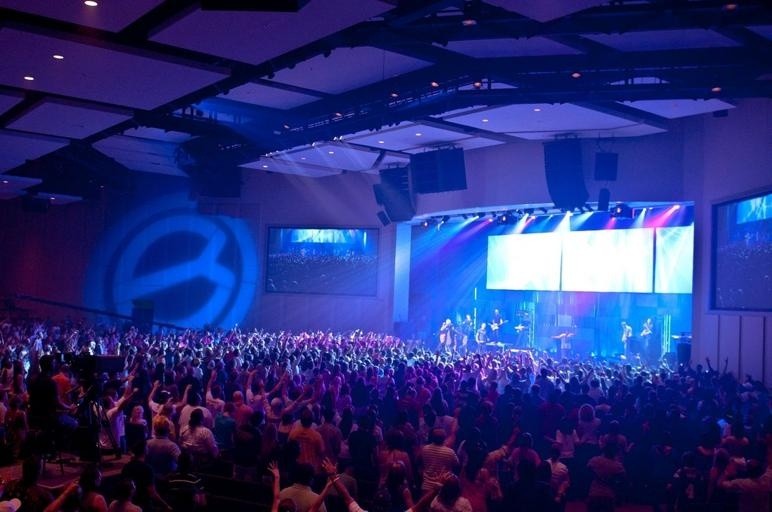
[491,321,509,331]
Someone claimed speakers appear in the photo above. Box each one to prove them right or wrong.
[377,211,392,227]
[378,162,416,222]
[542,138,589,209]
[595,152,620,181]
[677,340,690,373]
[374,182,384,209]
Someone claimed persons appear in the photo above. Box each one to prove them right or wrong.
[0,308,772,512]
[715,230,771,307]
[268,247,377,295]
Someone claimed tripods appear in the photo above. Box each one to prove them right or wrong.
[76,372,122,473]
